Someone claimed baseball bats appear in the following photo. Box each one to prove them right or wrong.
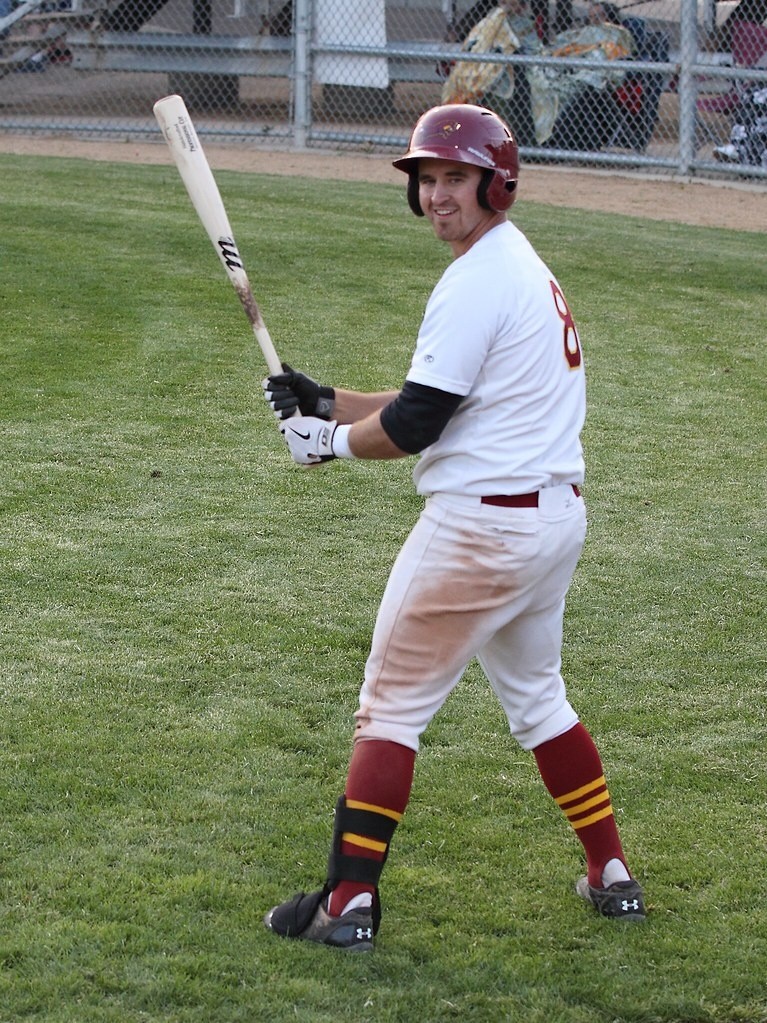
[153,94,304,420]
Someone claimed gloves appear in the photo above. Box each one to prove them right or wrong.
[279,415,339,464]
[262,361,335,422]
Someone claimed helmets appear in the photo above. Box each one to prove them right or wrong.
[392,103,521,217]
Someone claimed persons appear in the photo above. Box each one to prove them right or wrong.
[712,0,767,167]
[261,104,650,954]
[441,1,635,144]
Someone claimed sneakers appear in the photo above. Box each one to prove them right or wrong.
[713,144,747,164]
[263,889,374,955]
[575,875,646,922]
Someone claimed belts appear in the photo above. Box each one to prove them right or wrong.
[482,484,580,508]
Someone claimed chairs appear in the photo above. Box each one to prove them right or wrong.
[554,18,668,161]
